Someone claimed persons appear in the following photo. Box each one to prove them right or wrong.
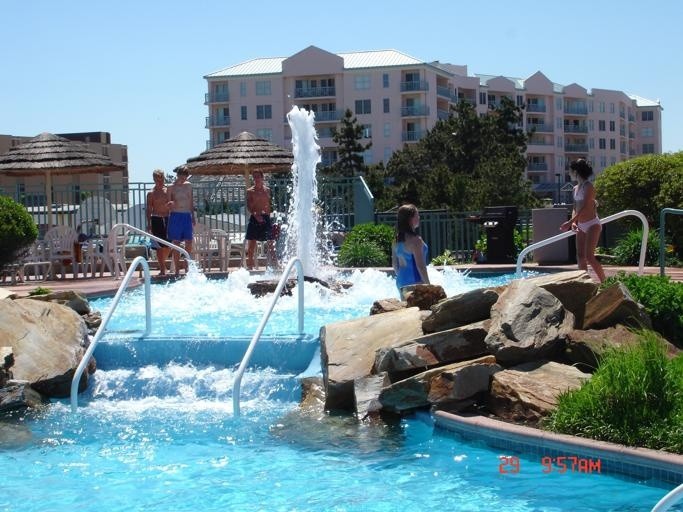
[558,158,606,283]
[391,204,430,300]
[245,170,284,271]
[146,165,197,276]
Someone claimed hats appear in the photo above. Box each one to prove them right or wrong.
[177,166,189,176]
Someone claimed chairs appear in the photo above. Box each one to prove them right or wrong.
[5,226,232,285]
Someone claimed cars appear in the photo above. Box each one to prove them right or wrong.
[115,236,148,257]
[447,250,486,263]
